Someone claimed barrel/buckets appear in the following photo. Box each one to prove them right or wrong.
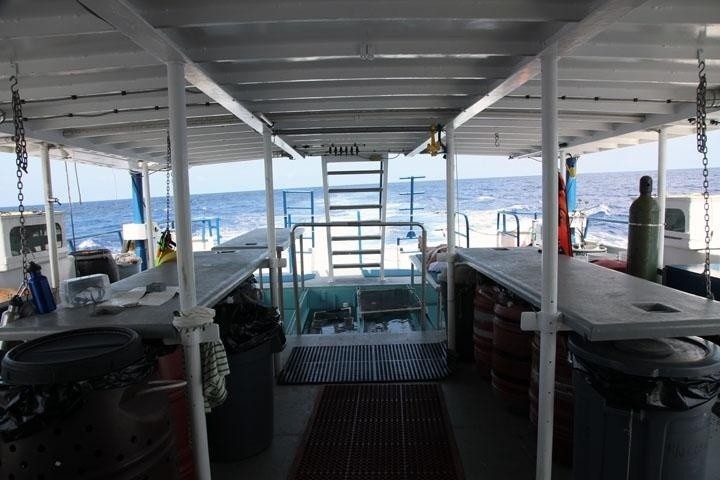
[490,300,527,394]
[471,285,495,378]
[529,334,584,461]
[157,341,196,478]
[204,341,275,464]
[70,248,119,283]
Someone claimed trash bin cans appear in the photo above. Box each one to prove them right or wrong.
[567,332,720,480]
[205,302,287,461]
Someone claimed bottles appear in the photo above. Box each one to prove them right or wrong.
[0,294,24,352]
[26,260,57,315]
